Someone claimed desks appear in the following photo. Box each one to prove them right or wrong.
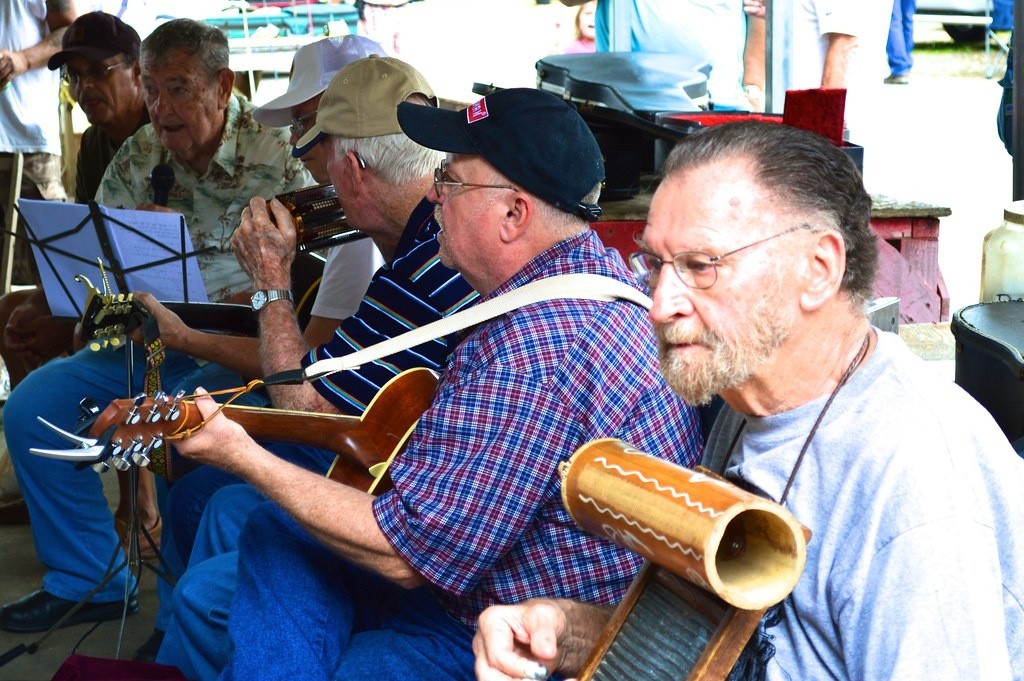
[589,188,951,324]
[898,321,956,382]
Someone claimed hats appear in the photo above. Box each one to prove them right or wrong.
[252,34,389,127]
[47,11,141,72]
[292,54,439,159]
[396,88,606,222]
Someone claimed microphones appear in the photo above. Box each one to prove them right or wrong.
[149,163,174,206]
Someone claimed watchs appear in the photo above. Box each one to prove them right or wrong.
[250,289,294,311]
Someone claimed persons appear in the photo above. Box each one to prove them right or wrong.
[0,0,1024,681]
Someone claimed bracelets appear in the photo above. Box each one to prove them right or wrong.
[741,84,762,100]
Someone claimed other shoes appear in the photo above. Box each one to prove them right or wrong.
[113,515,162,556]
[0,588,139,632]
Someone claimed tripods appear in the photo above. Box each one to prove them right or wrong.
[9,201,220,656]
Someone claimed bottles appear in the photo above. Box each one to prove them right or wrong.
[980,199,1024,303]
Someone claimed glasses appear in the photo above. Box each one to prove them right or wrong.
[59,61,123,87]
[290,110,317,136]
[627,220,818,289]
[433,160,519,198]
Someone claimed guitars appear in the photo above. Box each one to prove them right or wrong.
[71,367,441,499]
[77,279,323,353]
